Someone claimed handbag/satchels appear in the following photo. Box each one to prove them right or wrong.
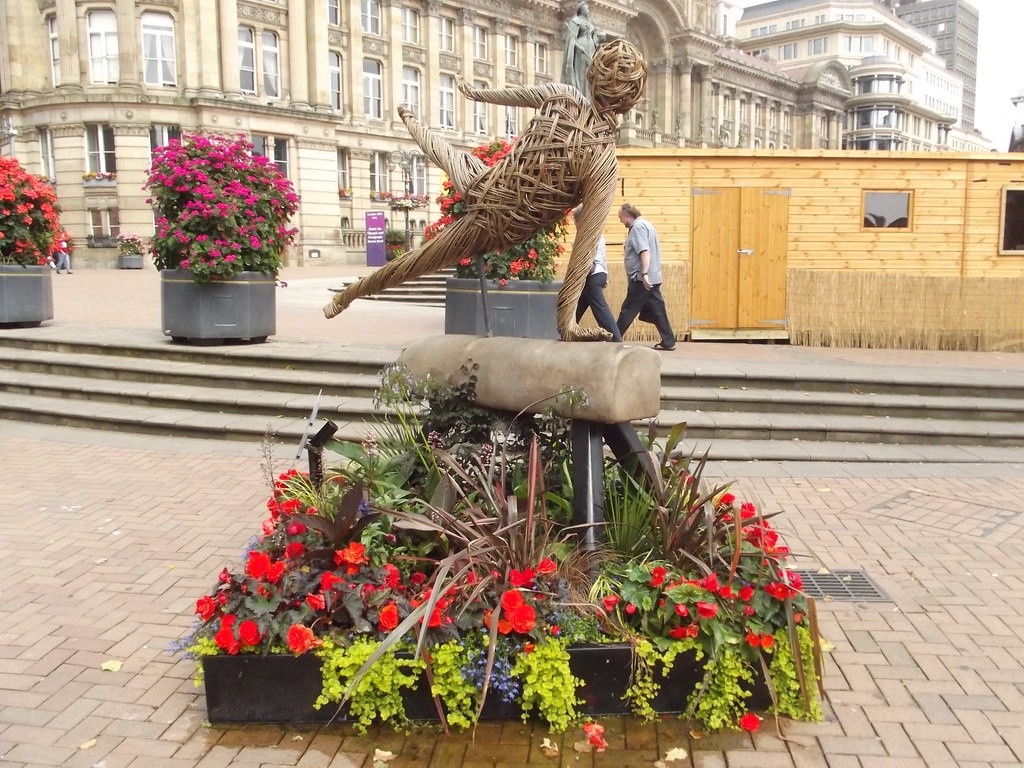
[639,310,655,323]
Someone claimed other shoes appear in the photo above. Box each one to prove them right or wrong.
[67,271,74,274]
[652,344,676,350]
[57,271,61,274]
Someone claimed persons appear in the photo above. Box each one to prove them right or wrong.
[55,232,73,274]
[562,1,606,103]
[616,203,677,350]
[572,203,623,343]
[323,38,649,343]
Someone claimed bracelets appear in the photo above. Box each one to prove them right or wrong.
[641,273,647,277]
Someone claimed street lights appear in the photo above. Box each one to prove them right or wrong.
[387,150,425,253]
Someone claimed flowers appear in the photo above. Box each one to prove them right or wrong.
[185,361,836,738]
[370,191,393,200]
[386,228,406,258]
[339,188,351,197]
[389,192,430,211]
[82,170,117,181]
[140,129,301,288]
[426,136,575,287]
[0,157,74,268]
[116,233,145,257]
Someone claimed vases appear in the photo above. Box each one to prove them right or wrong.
[339,196,352,201]
[0,265,54,323]
[371,198,391,201]
[386,251,397,262]
[120,255,144,268]
[202,643,800,723]
[161,268,276,337]
[446,276,564,344]
[83,179,117,188]
